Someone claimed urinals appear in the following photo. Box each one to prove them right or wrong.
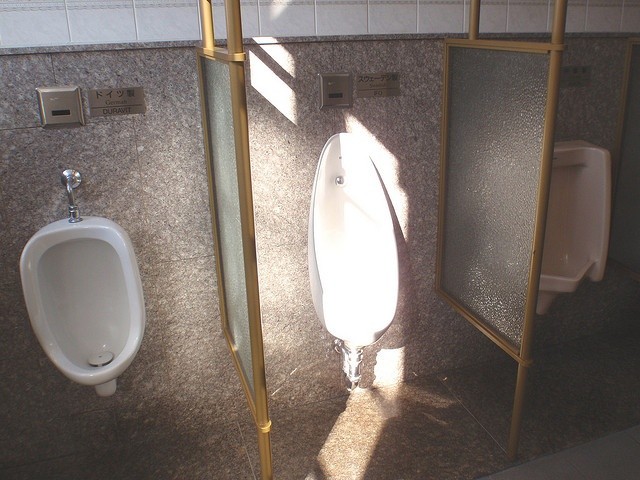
[19,215,147,397]
[308,132,400,347]
[533,139,612,315]
